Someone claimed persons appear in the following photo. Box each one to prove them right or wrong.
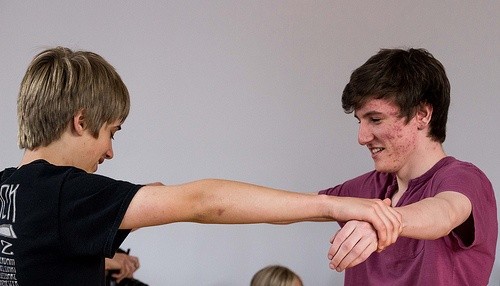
[0,46,404,286]
[102,249,140,286]
[251,265,303,286]
[272,48,499,286]
